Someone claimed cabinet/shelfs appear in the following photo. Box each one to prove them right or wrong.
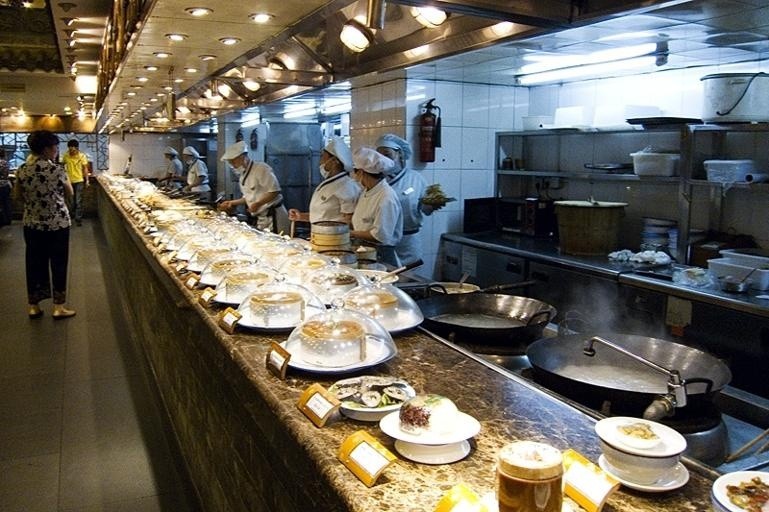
[494,126,686,267]
[679,123,769,272]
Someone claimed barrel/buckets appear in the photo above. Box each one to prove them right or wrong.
[554,203,628,258]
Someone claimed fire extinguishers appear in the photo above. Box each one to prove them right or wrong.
[420,98,441,162]
[236,129,243,142]
[250,128,257,150]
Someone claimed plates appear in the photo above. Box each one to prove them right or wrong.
[595,415,689,459]
[592,456,691,494]
[132,196,424,374]
[329,377,417,423]
[711,470,769,511]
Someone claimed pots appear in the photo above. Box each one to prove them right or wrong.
[524,329,732,432]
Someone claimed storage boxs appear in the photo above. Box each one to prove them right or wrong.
[631,153,681,176]
[719,249,768,265]
[703,160,756,183]
[706,257,768,292]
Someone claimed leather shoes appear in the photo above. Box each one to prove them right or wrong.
[28,309,44,319]
[53,308,77,321]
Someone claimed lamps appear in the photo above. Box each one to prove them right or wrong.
[339,14,376,53]
[411,4,452,30]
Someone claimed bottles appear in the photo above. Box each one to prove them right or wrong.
[496,441,564,512]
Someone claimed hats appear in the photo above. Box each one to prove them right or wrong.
[325,139,352,173]
[163,146,179,156]
[182,146,199,158]
[220,140,248,162]
[352,147,395,175]
[375,134,412,160]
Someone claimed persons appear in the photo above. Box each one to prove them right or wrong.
[288,137,361,229]
[12,129,76,319]
[350,147,404,268]
[59,138,90,227]
[0,148,9,180]
[216,140,291,236]
[182,145,211,192]
[373,134,446,276]
[157,146,183,188]
[24,131,53,164]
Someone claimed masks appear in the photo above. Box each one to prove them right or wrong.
[185,157,195,166]
[163,156,171,161]
[393,152,402,173]
[319,156,339,179]
[233,155,246,177]
[351,174,368,193]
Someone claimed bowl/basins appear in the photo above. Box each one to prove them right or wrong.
[597,439,682,484]
[432,281,483,299]
[414,291,557,355]
